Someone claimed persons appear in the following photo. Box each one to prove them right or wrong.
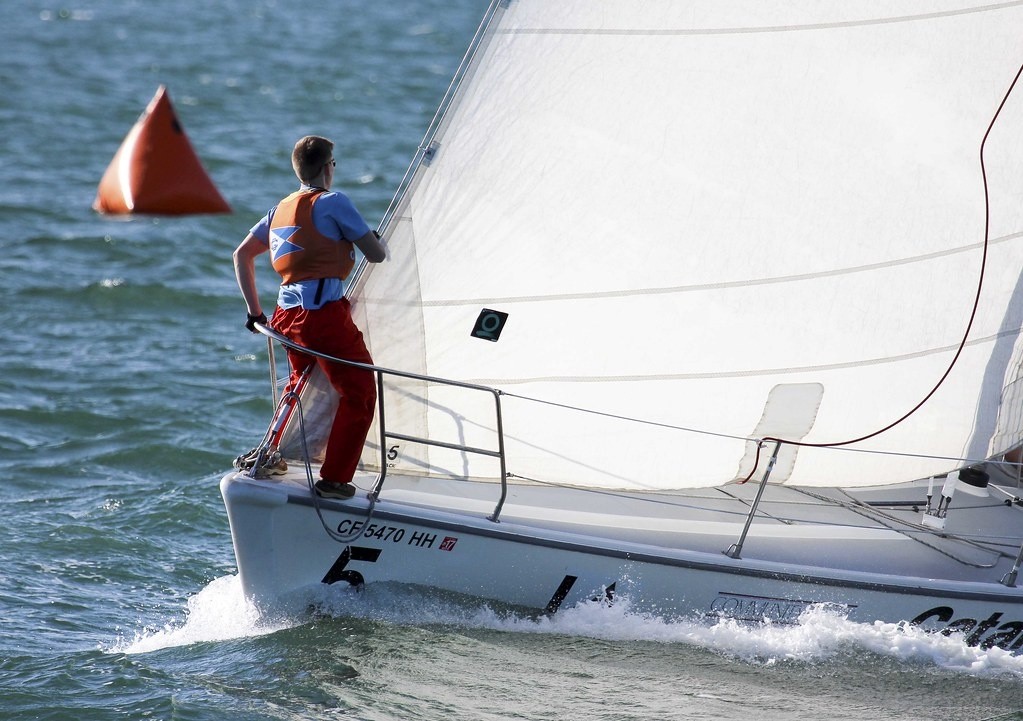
[972,443,1023,488]
[233,136,386,499]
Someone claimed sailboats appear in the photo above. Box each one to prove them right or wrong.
[218,0,1023,659]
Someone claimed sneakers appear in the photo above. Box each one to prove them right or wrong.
[313,480,355,499]
[268,458,287,476]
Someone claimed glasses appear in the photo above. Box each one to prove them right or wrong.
[325,159,335,167]
